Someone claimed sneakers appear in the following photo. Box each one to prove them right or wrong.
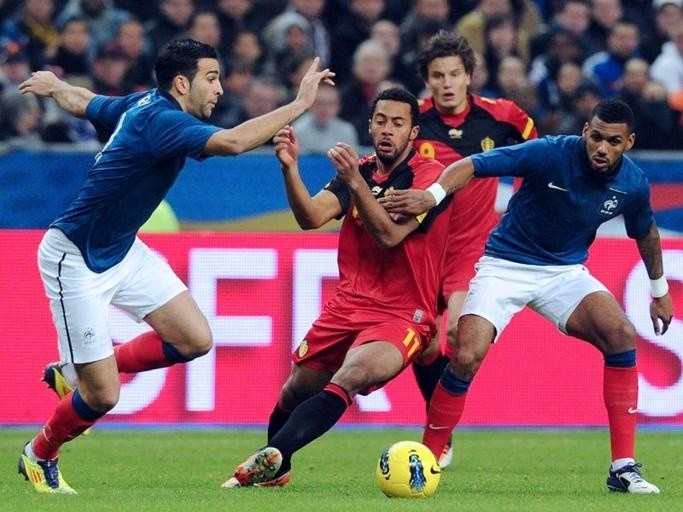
[18,441,77,494]
[42,361,91,435]
[438,446,453,468]
[221,447,291,488]
[607,462,659,494]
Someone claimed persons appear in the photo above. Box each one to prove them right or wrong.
[376,99,674,493]
[1,2,683,151]
[17,39,336,492]
[413,33,539,468]
[221,88,451,491]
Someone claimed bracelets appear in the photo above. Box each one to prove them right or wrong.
[426,183,447,206]
[649,275,670,298]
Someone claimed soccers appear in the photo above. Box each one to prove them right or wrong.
[376,440,442,497]
